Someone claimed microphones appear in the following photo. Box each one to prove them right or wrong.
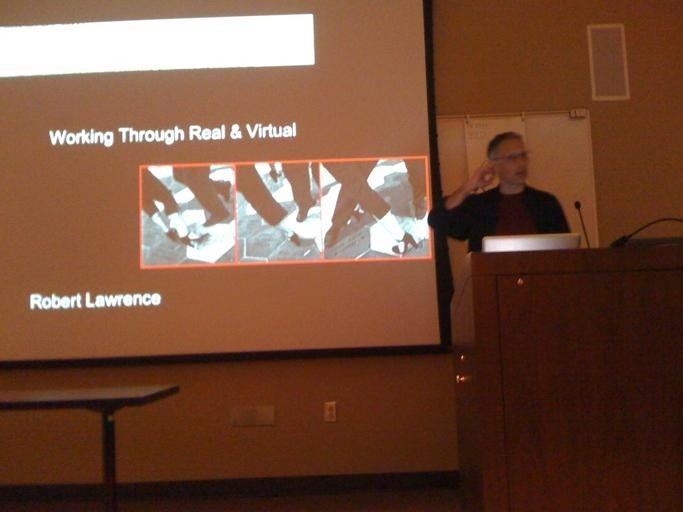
[611,217,683,247]
[574,200,590,249]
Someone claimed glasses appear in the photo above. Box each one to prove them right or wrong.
[492,152,528,162]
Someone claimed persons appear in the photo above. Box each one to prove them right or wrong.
[426,129,571,257]
[235,165,302,249]
[172,167,231,228]
[140,167,195,249]
[400,159,426,221]
[321,163,417,256]
[267,161,317,222]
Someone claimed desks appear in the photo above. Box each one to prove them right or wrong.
[0,385,179,512]
